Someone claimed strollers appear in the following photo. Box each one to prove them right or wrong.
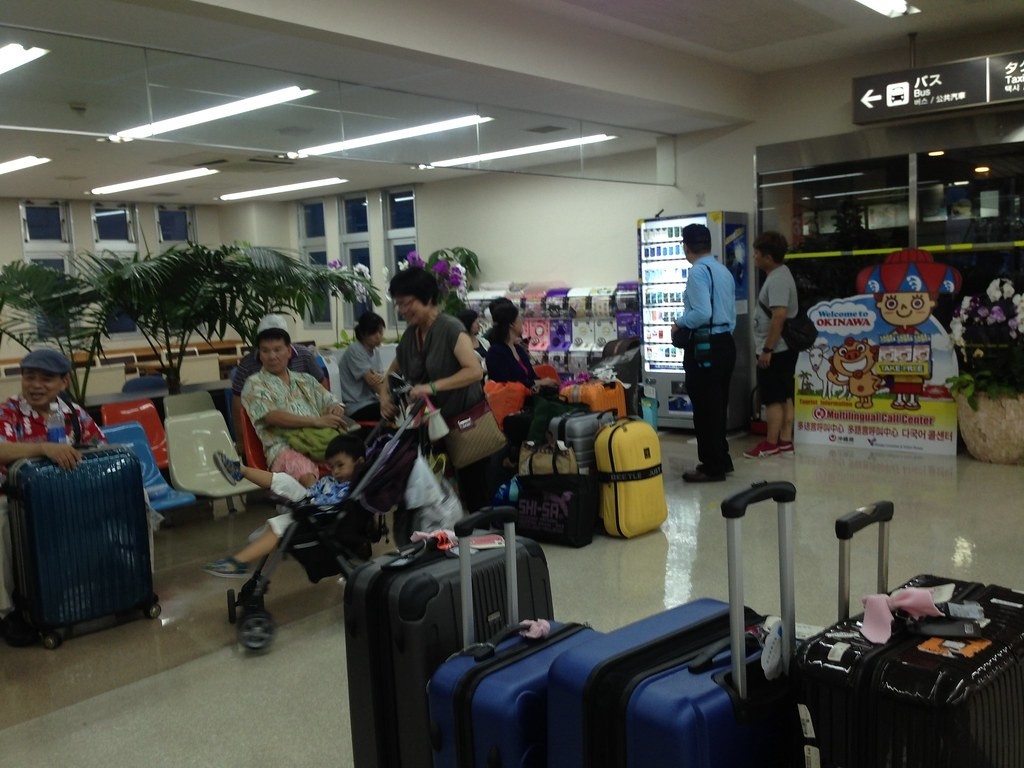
[227,390,429,652]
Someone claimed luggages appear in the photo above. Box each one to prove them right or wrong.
[547,371,668,538]
[6,445,161,649]
[345,480,1023,768]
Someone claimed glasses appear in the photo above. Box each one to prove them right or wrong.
[393,295,418,311]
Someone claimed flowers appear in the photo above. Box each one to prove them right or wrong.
[398,251,469,301]
[943,277,1024,411]
[328,259,371,303]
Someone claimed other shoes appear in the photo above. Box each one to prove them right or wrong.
[1,607,42,646]
[682,454,735,482]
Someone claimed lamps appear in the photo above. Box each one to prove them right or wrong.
[410,104,622,170]
[275,79,494,159]
[0,156,52,175]
[214,177,349,202]
[84,167,219,195]
[97,49,320,144]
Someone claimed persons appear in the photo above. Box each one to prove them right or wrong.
[743,230,799,458]
[380,267,507,506]
[671,224,737,483]
[338,311,386,422]
[202,433,367,579]
[457,299,561,440]
[0,350,110,647]
[241,328,349,514]
[232,314,330,409]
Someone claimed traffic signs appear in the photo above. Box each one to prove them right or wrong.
[849,50,1024,125]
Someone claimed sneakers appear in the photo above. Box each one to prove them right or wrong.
[743,439,794,461]
[213,451,245,486]
[203,555,250,579]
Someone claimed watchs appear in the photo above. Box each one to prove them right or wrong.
[335,402,347,409]
[763,346,773,353]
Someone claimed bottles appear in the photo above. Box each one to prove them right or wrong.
[642,227,666,242]
[647,329,657,341]
[647,345,652,359]
[46,402,66,444]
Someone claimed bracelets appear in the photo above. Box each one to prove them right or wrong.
[674,320,681,329]
[428,381,437,397]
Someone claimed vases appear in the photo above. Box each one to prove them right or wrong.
[321,342,398,372]
[957,393,1024,465]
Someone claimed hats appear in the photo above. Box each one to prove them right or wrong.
[20,350,71,374]
[256,313,290,337]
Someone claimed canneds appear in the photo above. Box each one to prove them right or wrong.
[642,227,690,357]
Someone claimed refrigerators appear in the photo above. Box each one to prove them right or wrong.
[637,211,747,430]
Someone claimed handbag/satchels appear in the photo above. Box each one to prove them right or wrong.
[683,328,726,379]
[503,406,530,463]
[418,391,451,443]
[516,432,597,548]
[485,379,532,432]
[759,299,819,353]
[272,412,349,462]
[526,394,576,444]
[443,400,507,470]
[355,418,466,550]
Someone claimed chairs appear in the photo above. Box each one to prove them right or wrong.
[0,363,22,378]
[95,352,141,379]
[160,346,199,380]
[240,407,266,472]
[164,409,261,498]
[100,421,196,509]
[162,390,214,415]
[226,367,237,440]
[122,377,168,393]
[102,400,169,468]
[235,343,252,365]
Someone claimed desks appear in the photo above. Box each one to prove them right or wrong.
[129,355,240,376]
[84,379,227,426]
[0,340,245,365]
